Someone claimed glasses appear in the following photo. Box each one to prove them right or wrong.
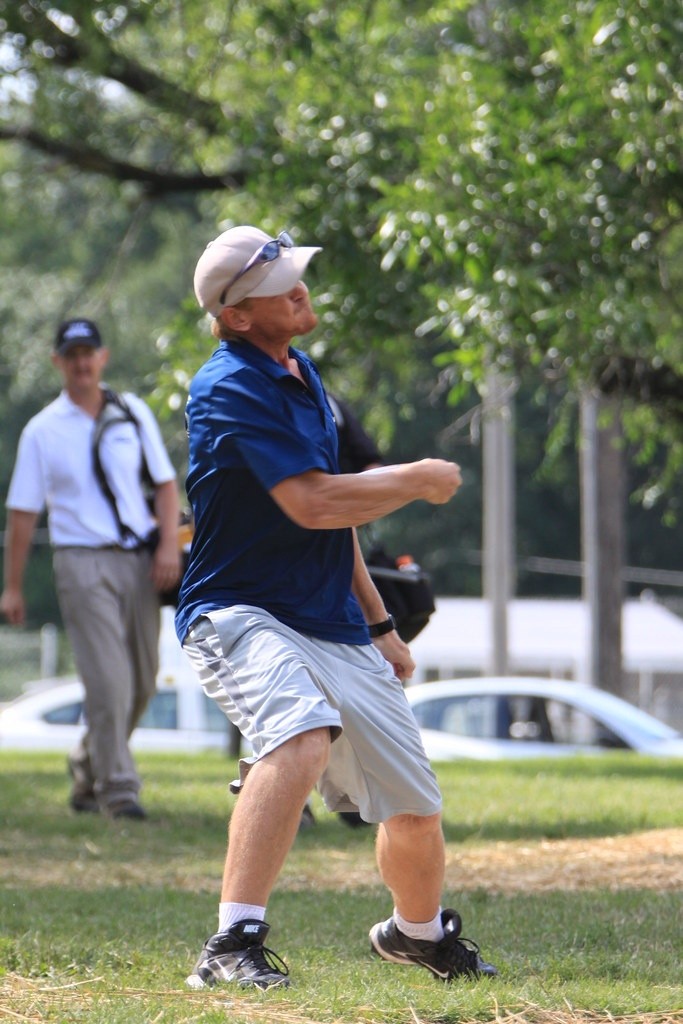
[218,232,293,305]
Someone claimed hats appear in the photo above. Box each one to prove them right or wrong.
[56,318,101,357]
[193,225,323,317]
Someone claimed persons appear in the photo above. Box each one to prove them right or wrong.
[172,226,504,999]
[0,318,185,826]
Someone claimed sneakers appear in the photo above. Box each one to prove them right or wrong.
[370,909,496,983]
[186,919,290,989]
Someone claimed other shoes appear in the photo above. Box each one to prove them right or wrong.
[68,793,98,813]
[102,797,142,822]
[339,810,371,830]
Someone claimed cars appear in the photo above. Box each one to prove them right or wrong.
[401,675,683,759]
[0,665,254,756]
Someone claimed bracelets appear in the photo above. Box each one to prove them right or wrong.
[367,612,396,637]
